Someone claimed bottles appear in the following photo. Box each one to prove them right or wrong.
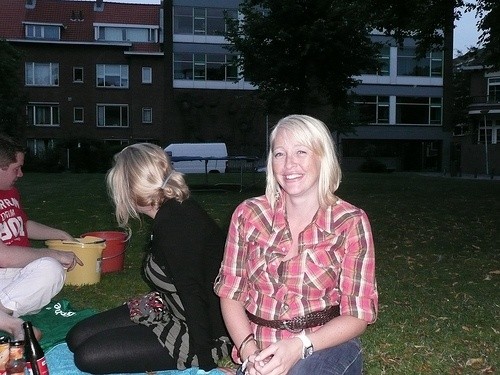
[23,321,49,375]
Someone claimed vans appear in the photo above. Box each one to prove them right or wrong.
[164,143,229,176]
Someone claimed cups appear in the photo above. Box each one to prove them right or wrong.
[10,341,25,360]
[0,336,9,372]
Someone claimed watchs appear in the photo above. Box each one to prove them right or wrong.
[295,335,314,359]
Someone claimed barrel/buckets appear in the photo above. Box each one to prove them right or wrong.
[80,231,131,273]
[44,236,106,287]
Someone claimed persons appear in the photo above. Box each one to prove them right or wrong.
[66,142,226,375]
[213,114,378,375]
[0,137,83,341]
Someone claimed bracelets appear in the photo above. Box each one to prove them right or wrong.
[237,333,259,354]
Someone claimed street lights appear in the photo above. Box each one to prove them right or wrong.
[480,108,490,175]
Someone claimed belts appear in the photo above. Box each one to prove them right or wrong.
[246,305,340,333]
